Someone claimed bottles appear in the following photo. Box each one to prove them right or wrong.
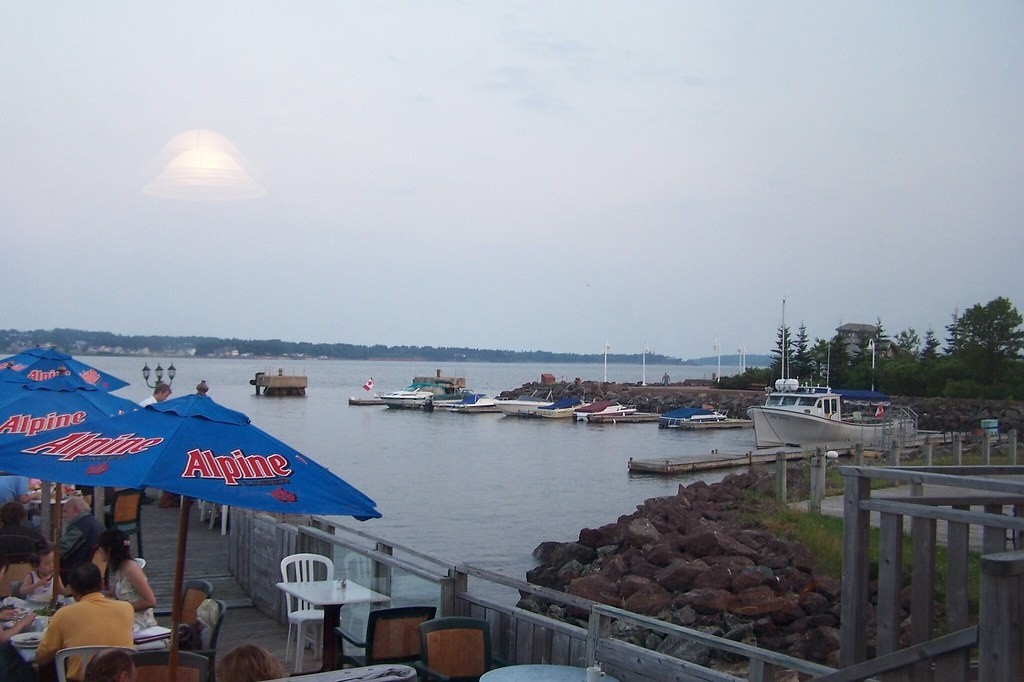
[55,595,65,613]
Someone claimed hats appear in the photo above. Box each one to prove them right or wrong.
[92,530,130,547]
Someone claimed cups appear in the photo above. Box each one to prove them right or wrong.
[34,616,48,633]
[586,667,605,682]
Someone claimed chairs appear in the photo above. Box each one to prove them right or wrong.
[336,605,438,671]
[188,598,228,682]
[279,553,343,674]
[134,650,210,682]
[109,486,145,558]
[0,534,44,598]
[133,557,147,569]
[414,616,491,682]
[180,579,214,625]
[56,644,138,682]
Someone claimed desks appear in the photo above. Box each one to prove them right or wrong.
[274,579,391,682]
[479,664,621,682]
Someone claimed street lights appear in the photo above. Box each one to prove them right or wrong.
[641,342,649,387]
[867,338,876,393]
[713,337,721,384]
[737,341,748,376]
[603,339,610,382]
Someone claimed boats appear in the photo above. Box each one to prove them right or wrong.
[377,382,445,410]
[431,391,502,413]
[493,389,556,418]
[571,400,638,421]
[534,397,589,419]
[746,376,917,450]
[658,407,729,428]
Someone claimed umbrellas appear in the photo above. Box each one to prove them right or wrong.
[0,343,383,682]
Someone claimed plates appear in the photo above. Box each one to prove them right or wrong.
[28,593,53,604]
[10,632,42,645]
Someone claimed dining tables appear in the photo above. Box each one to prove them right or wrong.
[29,497,66,505]
[0,594,171,682]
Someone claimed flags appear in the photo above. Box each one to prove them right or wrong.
[875,403,884,416]
[363,379,374,392]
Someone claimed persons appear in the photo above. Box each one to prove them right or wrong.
[217,646,285,682]
[57,497,106,588]
[83,648,137,682]
[140,384,172,408]
[20,546,72,601]
[98,528,156,628]
[0,502,47,552]
[65,485,116,528]
[34,561,136,682]
[0,475,42,507]
[0,550,37,669]
